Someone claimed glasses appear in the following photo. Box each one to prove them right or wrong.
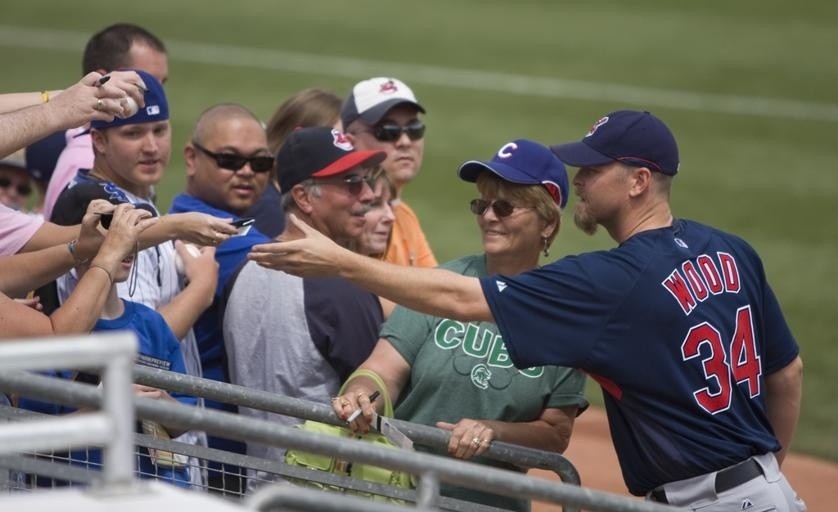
[351,119,426,141]
[470,198,538,217]
[0,177,32,197]
[305,175,375,193]
[192,141,275,173]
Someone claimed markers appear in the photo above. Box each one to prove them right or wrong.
[93,75,110,88]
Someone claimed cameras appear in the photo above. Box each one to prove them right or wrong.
[99,200,159,232]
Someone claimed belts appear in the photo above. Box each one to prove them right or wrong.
[651,460,763,506]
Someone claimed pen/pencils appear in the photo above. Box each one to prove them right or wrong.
[344,389,381,426]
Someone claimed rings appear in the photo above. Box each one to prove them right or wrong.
[484,440,491,445]
[211,239,217,246]
[356,392,369,407]
[473,437,480,447]
[341,400,351,408]
[329,396,339,405]
[97,96,102,111]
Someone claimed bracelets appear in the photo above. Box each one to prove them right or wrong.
[69,239,82,267]
[41,88,49,103]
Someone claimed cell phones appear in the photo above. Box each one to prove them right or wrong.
[228,218,256,231]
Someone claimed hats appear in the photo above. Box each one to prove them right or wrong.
[549,111,680,175]
[2,150,26,172]
[73,70,169,138]
[458,140,567,211]
[341,77,426,136]
[277,126,387,195]
[51,181,157,228]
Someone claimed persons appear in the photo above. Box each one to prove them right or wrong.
[172,101,278,484]
[343,77,439,269]
[336,136,586,512]
[223,128,383,491]
[45,23,170,224]
[246,88,343,232]
[34,71,209,495]
[0,71,147,158]
[248,112,807,512]
[2,144,41,212]
[0,198,157,338]
[0,207,239,247]
[19,182,196,489]
